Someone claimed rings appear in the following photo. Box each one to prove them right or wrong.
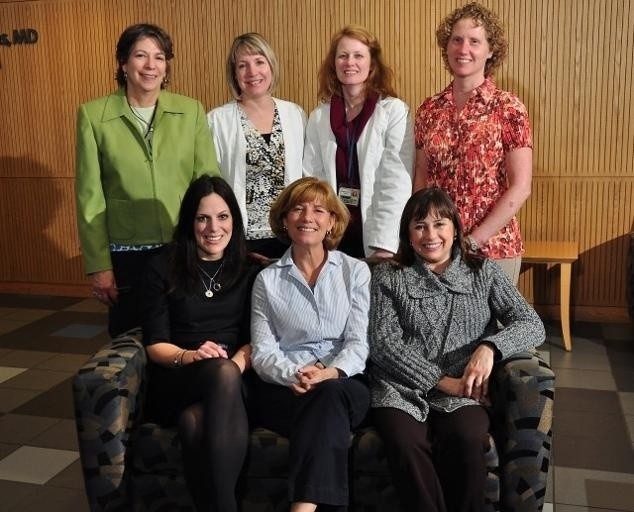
[92,293,98,296]
[97,295,101,298]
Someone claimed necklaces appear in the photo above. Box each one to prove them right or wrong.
[193,259,227,299]
[130,106,150,127]
[196,262,222,292]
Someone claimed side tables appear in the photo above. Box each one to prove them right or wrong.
[520,240,578,354]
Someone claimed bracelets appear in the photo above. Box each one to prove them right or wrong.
[466,235,479,251]
[171,348,188,368]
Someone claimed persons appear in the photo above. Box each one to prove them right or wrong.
[200,30,311,268]
[139,173,274,509]
[296,24,418,268]
[73,24,223,339]
[366,186,549,507]
[410,1,534,333]
[246,175,372,510]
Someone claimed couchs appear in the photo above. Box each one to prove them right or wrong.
[72,258,556,512]
